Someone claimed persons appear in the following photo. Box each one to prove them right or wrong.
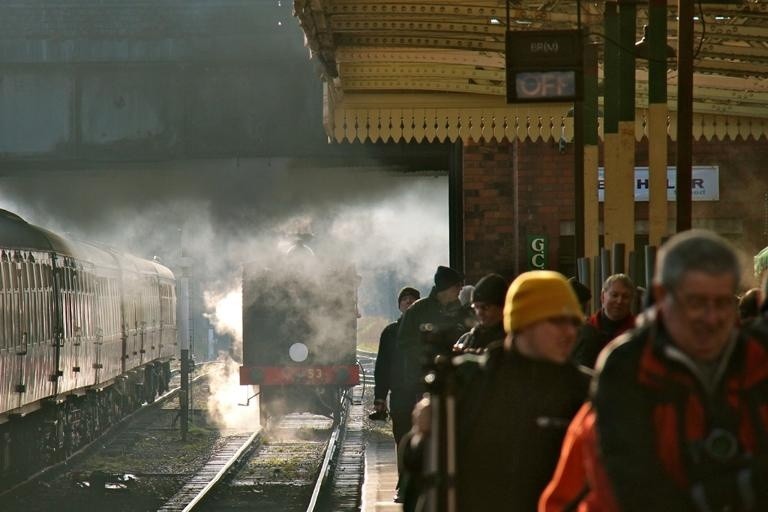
[369,229,768,511]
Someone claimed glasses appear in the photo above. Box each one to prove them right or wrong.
[472,303,490,310]
[665,287,739,313]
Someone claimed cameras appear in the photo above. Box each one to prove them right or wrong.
[369,408,389,421]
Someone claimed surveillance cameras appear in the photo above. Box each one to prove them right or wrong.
[557,137,567,155]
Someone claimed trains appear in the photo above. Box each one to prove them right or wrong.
[239,228,363,433]
[0,207,177,493]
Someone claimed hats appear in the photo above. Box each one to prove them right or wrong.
[398,286,420,308]
[471,271,585,333]
[434,266,464,291]
